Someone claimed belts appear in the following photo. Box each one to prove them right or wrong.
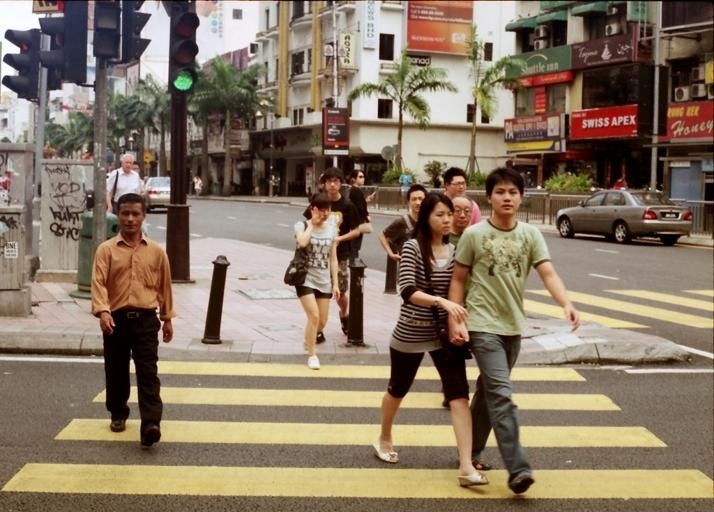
[114,311,152,319]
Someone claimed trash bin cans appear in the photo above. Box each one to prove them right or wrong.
[70,212,121,298]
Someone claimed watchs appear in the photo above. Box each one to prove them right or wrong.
[433,295,441,305]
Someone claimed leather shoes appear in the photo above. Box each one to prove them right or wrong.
[110,418,125,432]
[139,424,161,451]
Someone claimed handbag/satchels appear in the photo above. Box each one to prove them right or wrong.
[107,197,114,208]
[284,247,309,286]
[437,326,472,364]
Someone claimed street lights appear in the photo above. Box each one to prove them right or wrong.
[256,100,276,197]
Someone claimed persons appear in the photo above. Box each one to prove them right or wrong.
[193,176,204,198]
[446,166,581,496]
[612,174,625,190]
[347,169,376,279]
[447,195,475,310]
[90,192,176,449]
[380,166,482,264]
[369,191,492,490]
[293,192,342,371]
[302,167,365,346]
[105,150,149,215]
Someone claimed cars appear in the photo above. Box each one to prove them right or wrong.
[555,186,691,247]
[142,176,170,212]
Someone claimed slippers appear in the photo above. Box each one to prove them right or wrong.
[316,332,325,344]
[458,471,489,487]
[509,476,535,493]
[459,456,492,469]
[372,441,399,463]
[339,310,349,335]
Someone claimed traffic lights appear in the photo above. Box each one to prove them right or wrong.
[169,13,200,95]
[0,25,37,98]
[127,0,154,58]
[92,1,121,60]
[39,0,87,79]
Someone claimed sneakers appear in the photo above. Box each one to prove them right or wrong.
[308,354,320,370]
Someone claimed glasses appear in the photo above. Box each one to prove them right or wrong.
[454,208,471,216]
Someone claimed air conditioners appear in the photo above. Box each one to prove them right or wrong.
[671,64,713,103]
[607,6,620,17]
[531,28,547,51]
[604,22,619,36]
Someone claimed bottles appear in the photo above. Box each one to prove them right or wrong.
[601,44,612,60]
